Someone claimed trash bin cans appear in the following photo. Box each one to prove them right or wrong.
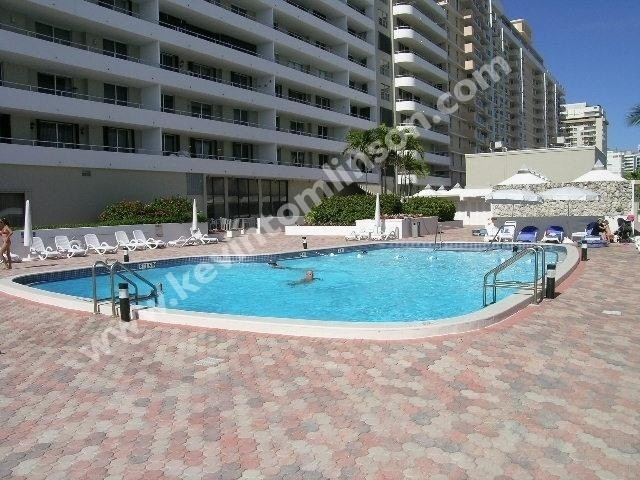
[412,221,420,238]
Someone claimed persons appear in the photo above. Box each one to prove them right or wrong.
[0,215,13,270]
[597,218,611,241]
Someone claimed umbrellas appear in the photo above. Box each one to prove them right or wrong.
[497,169,550,197]
[485,187,538,221]
[192,199,197,233]
[571,169,629,215]
[539,186,597,235]
[23,199,33,257]
[374,193,381,234]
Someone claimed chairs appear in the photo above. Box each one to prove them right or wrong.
[1,212,257,258]
[344,225,404,241]
[484,215,605,249]
